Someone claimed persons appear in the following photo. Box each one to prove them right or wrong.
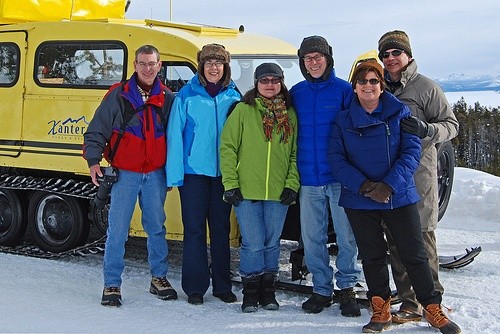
[166,43,238,306]
[378,30,460,324]
[326,58,461,334]
[220,63,300,313]
[290,36,356,317]
[81,46,179,308]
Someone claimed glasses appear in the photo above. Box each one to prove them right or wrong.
[259,78,282,84]
[303,53,323,62]
[206,62,223,68]
[138,62,158,67]
[357,79,379,85]
[383,50,405,58]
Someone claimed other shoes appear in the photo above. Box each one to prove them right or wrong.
[339,288,361,317]
[391,311,422,323]
[188,294,203,304]
[302,293,333,314]
[213,291,237,302]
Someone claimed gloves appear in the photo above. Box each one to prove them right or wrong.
[363,182,393,202]
[280,188,298,206]
[401,115,427,139]
[361,180,390,204]
[224,188,243,207]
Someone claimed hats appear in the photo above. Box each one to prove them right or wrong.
[378,30,412,62]
[254,63,285,85]
[197,44,232,87]
[298,35,334,82]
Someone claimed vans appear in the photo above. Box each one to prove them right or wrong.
[0,0,455,257]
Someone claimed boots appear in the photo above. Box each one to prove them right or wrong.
[259,273,280,310]
[407,298,461,334]
[362,295,392,333]
[240,276,259,312]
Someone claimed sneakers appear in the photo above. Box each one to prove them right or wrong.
[100,287,123,306]
[150,275,178,300]
